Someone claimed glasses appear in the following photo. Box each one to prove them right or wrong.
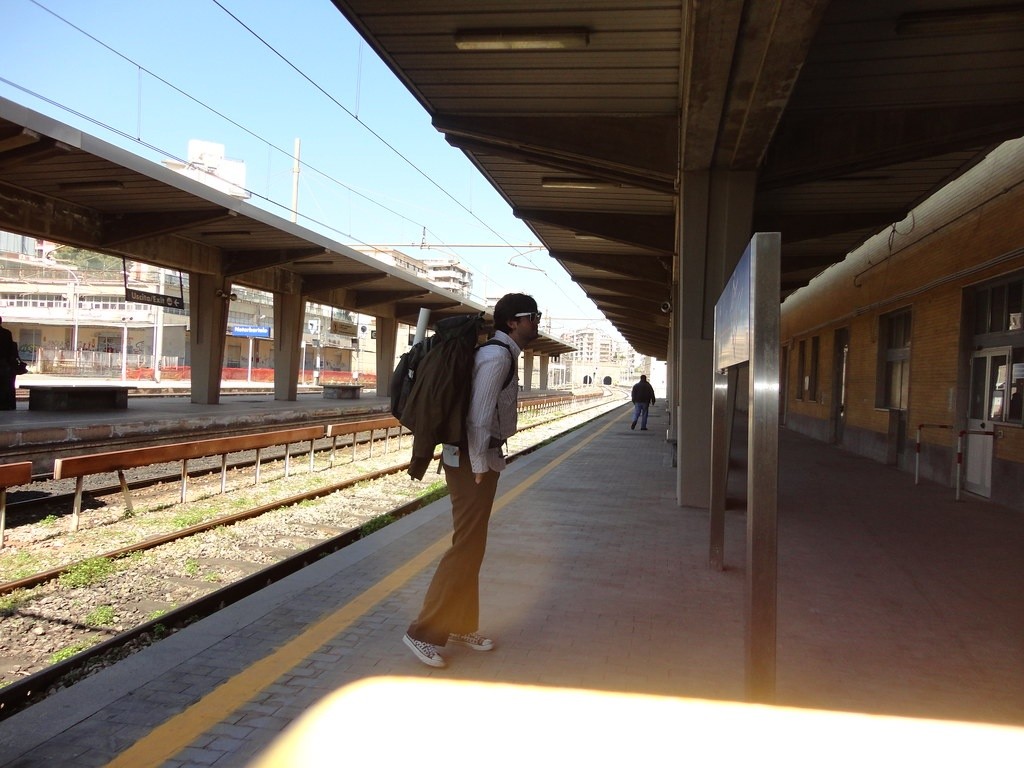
[515,313,541,322]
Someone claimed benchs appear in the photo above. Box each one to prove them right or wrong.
[319,384,364,400]
[18,384,137,411]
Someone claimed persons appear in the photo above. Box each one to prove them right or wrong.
[402,294,542,668]
[631,375,655,430]
[0,317,17,410]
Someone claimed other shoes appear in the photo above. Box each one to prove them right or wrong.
[447,629,495,651]
[402,632,447,668]
[631,421,637,430]
[641,428,647,430]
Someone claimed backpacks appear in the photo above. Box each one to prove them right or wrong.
[391,312,515,480]
